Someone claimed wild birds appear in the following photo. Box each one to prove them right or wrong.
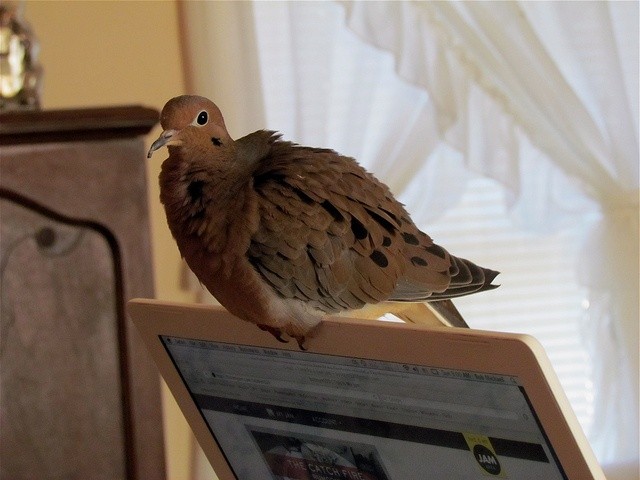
[146,93,503,353]
